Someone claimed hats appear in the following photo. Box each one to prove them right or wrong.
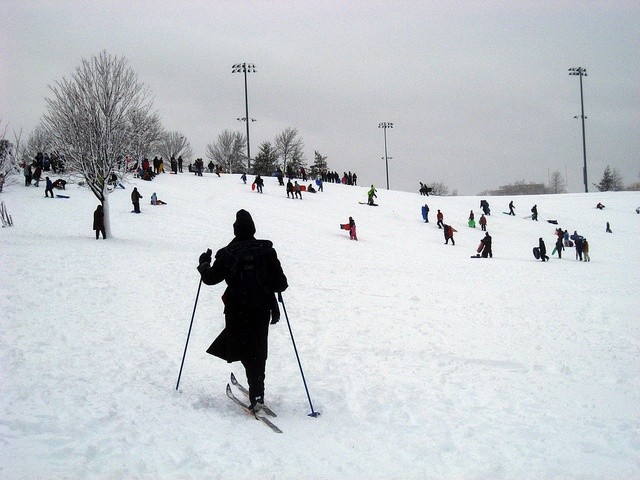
[233,209,256,235]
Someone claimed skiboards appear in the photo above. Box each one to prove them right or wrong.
[226,372,283,433]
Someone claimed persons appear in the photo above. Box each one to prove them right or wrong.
[186,157,226,177]
[481,199,493,215]
[468,209,474,227]
[422,203,429,224]
[443,224,456,244]
[241,172,247,184]
[255,175,262,192]
[285,164,378,207]
[436,210,448,229]
[111,153,183,184]
[529,227,591,265]
[509,200,515,216]
[415,181,435,197]
[346,216,356,240]
[93,206,107,241]
[151,192,167,206]
[21,149,67,199]
[481,232,492,259]
[274,165,284,185]
[132,188,141,213]
[531,204,537,220]
[198,208,288,411]
[596,201,603,211]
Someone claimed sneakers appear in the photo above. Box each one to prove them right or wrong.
[249,395,265,413]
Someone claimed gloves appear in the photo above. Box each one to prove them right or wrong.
[199,252,212,264]
